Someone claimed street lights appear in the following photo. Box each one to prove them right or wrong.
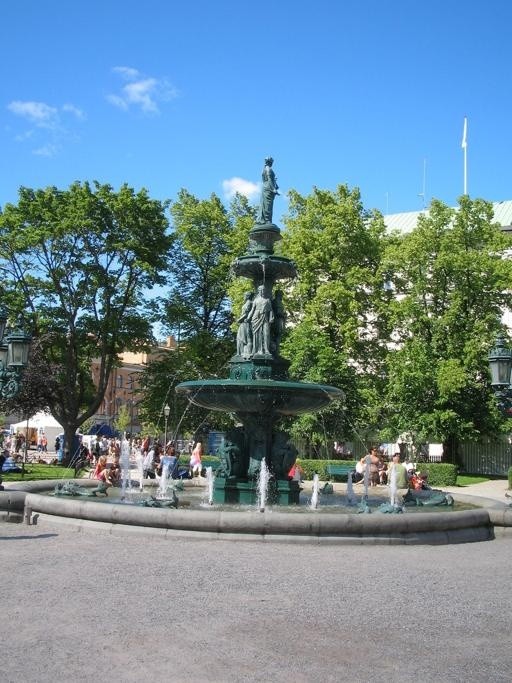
[163,403,171,457]
[24,406,30,463]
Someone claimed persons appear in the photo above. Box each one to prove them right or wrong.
[0,431,202,485]
[254,156,281,224]
[354,445,431,490]
[236,284,288,356]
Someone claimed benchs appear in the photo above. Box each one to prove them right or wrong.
[325,465,357,482]
